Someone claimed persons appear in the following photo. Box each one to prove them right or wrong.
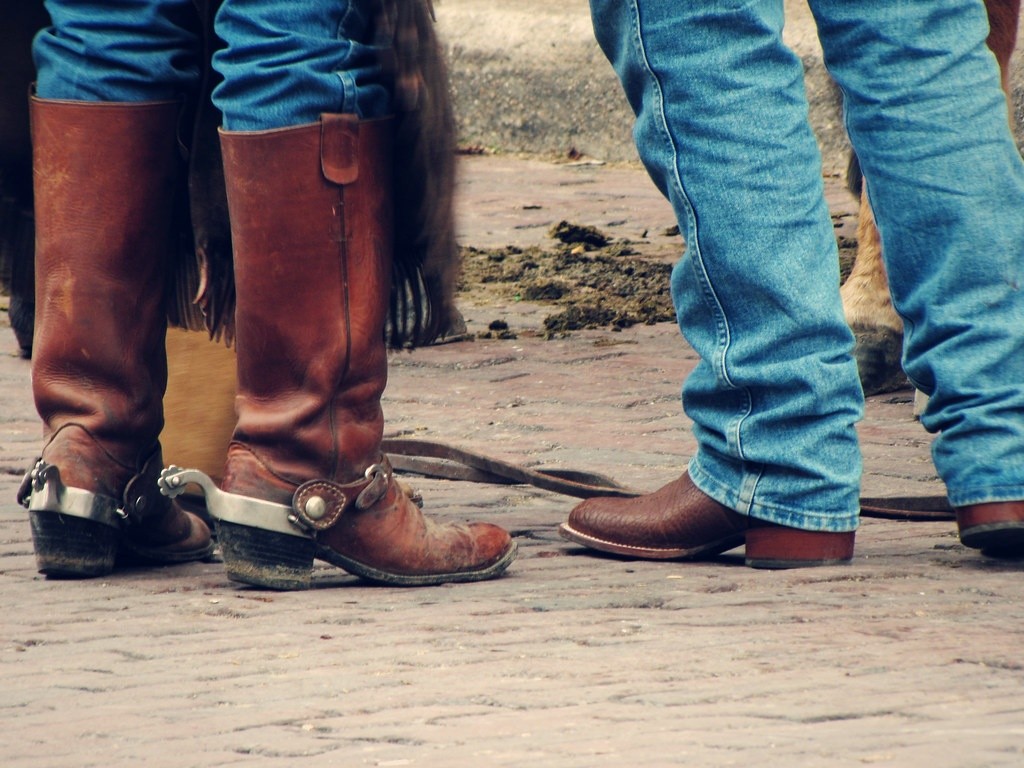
[561,0,1024,569]
[19,0,517,589]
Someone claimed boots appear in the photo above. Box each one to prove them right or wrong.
[16,81,214,578]
[158,112,518,590]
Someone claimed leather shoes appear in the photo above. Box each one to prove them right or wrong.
[955,500,1024,560]
[558,468,855,569]
